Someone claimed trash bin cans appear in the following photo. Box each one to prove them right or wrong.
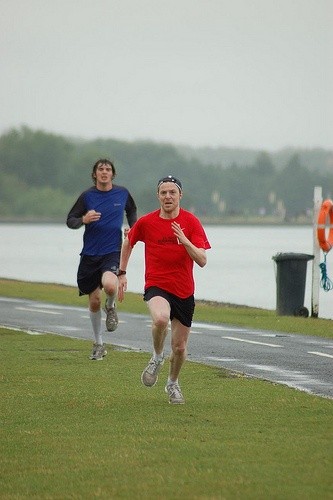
[271,251,315,318]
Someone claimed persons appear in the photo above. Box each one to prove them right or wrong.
[118,176,211,404]
[66,158,138,360]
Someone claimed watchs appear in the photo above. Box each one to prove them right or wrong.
[118,269,126,277]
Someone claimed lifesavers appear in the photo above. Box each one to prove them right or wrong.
[318,199,333,251]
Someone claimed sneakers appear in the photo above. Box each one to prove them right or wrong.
[104,299,119,331]
[164,382,185,405]
[91,344,107,360]
[141,357,165,388]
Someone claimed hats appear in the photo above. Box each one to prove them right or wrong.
[158,177,182,192]
